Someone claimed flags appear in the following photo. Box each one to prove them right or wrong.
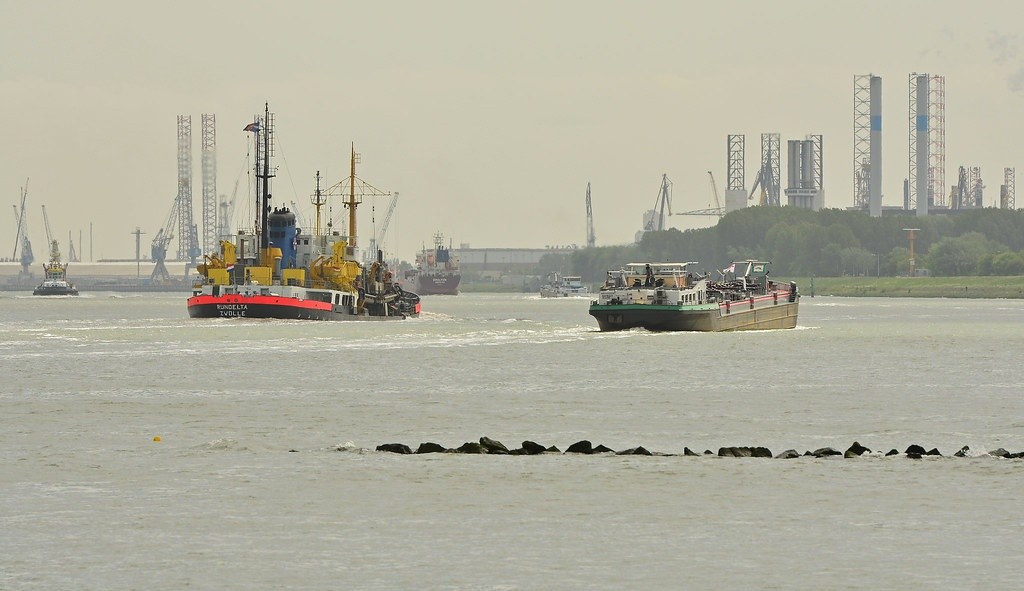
[227,263,234,272]
[723,263,735,273]
[243,123,259,133]
[752,264,764,272]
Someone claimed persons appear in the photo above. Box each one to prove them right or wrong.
[646,264,652,276]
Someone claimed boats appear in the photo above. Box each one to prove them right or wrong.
[412,230,461,295]
[186,100,421,321]
[589,258,801,331]
[539,271,590,299]
[33,240,79,297]
